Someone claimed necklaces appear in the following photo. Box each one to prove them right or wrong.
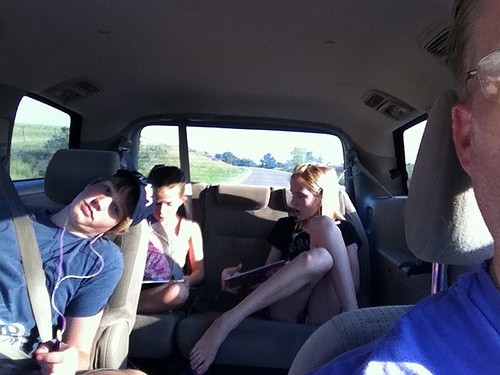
[292,223,309,239]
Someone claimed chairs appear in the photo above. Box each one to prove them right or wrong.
[28,147,148,370]
[286,88,494,375]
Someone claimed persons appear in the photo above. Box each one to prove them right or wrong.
[188,162,363,375]
[307,0,500,375]
[0,169,155,375]
[137,163,205,313]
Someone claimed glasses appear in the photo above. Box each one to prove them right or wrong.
[460,48,500,104]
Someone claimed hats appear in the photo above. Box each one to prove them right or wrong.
[117,168,155,227]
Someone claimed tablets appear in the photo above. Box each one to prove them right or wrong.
[141,279,186,292]
[223,260,286,290]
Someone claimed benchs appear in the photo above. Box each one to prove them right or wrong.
[130,182,371,368]
